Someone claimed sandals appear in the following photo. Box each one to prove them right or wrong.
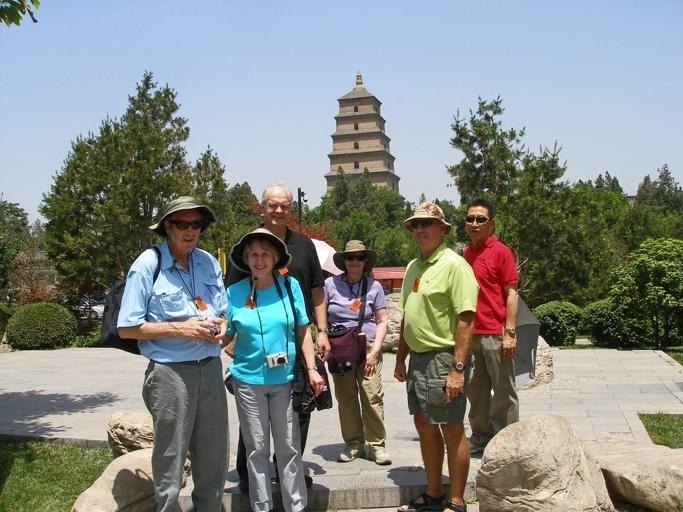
[397,493,467,512]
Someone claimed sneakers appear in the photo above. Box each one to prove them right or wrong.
[369,447,391,464]
[338,446,365,462]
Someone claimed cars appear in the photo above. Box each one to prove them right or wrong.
[76,296,104,320]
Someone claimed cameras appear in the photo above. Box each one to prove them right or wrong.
[209,328,217,336]
[330,359,352,376]
[266,352,288,368]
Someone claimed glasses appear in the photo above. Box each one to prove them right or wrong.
[346,255,365,261]
[411,220,433,227]
[168,220,202,229]
[466,217,488,222]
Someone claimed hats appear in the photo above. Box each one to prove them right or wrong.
[229,228,292,273]
[403,203,452,235]
[148,196,215,238]
[333,240,375,270]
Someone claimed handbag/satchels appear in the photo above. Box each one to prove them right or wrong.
[328,325,365,373]
[225,353,332,412]
[99,280,140,354]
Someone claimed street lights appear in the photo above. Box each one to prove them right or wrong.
[294,185,309,233]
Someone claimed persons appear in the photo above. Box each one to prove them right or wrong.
[464,201,519,455]
[215,227,324,512]
[116,195,227,511]
[323,239,393,466]
[223,183,334,494]
[394,201,481,512]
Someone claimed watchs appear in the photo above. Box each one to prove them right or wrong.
[452,361,465,371]
[504,325,516,334]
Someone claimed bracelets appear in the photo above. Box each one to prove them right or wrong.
[305,366,317,370]
